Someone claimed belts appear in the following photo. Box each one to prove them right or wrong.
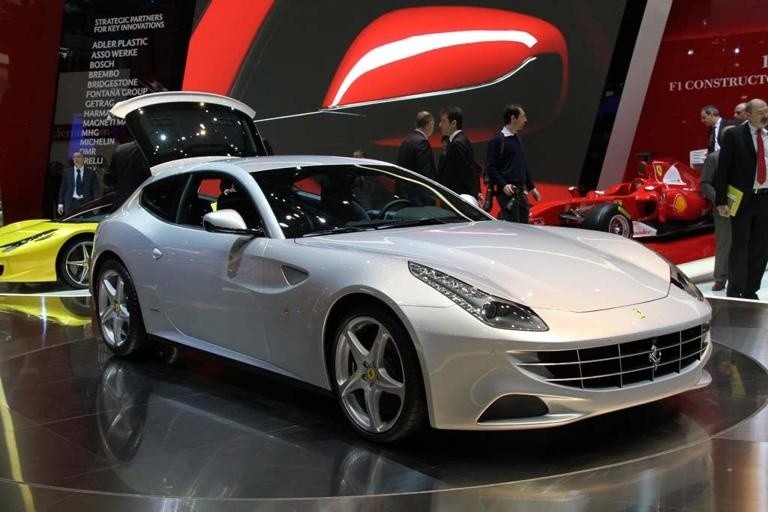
[753,188,767,195]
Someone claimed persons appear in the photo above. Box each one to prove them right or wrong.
[701,125,736,291]
[438,106,485,221]
[700,103,741,155]
[734,101,750,125]
[485,104,541,225]
[57,150,102,221]
[351,150,369,184]
[716,99,768,299]
[395,110,436,208]
[437,134,451,182]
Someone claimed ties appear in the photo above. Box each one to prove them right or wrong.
[710,127,715,153]
[75,168,82,196]
[754,128,766,184]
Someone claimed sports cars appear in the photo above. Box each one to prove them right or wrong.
[524,153,715,242]
[93,354,719,511]
[1,195,129,294]
[0,285,96,330]
[87,89,715,447]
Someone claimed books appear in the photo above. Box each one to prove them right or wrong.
[727,185,743,217]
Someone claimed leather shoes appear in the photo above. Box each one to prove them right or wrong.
[712,281,725,291]
